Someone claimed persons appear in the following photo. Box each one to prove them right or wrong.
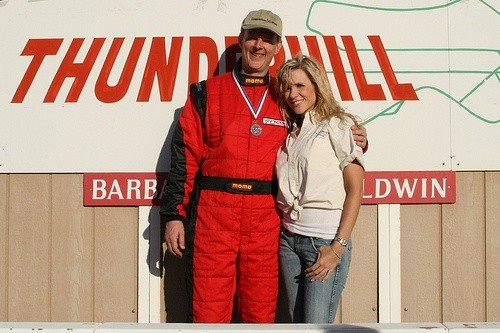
[158,8,368,325]
[276,52,366,324]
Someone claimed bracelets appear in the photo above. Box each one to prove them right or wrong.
[331,245,341,260]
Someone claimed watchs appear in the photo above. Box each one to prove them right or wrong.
[334,236,348,247]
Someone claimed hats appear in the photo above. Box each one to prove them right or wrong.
[241,11,283,41]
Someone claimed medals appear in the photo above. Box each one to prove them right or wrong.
[250,122,262,137]
